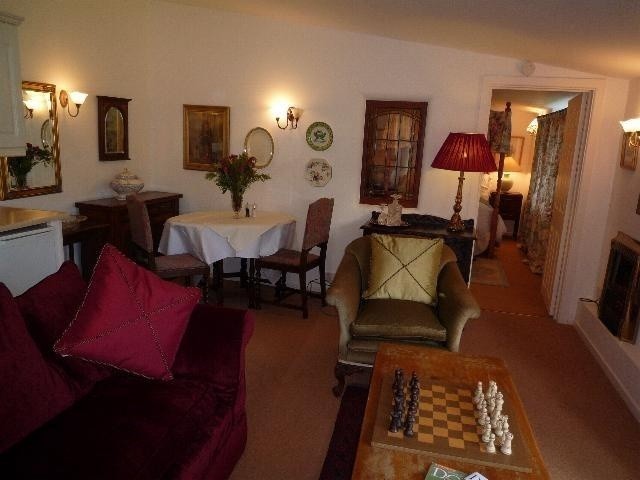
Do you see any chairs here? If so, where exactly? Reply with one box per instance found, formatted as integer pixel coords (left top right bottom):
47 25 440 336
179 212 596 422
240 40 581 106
250 197 335 319
127 194 209 301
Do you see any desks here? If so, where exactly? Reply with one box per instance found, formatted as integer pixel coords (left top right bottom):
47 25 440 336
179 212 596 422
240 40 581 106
166 209 297 307
56 212 111 273
362 215 475 291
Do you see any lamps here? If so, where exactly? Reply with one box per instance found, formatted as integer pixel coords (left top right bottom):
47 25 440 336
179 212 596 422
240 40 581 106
269 94 302 129
495 156 523 192
60 89 88 118
429 132 498 224
618 117 640 145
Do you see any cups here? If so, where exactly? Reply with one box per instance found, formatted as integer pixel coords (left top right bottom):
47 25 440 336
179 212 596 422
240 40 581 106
378 204 388 224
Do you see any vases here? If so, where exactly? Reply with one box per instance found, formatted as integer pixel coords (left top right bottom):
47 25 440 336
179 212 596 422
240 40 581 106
18 173 27 189
231 198 242 220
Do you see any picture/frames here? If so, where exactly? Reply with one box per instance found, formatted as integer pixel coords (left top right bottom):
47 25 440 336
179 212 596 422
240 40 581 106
305 121 334 152
0 81 63 201
358 98 428 209
302 158 334 188
181 104 229 171
620 132 640 171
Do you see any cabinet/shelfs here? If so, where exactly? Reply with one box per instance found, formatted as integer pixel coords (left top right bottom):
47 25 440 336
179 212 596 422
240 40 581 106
0 7 32 158
0 205 64 298
77 180 183 279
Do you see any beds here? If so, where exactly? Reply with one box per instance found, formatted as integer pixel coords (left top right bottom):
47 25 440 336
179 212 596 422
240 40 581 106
462 173 507 258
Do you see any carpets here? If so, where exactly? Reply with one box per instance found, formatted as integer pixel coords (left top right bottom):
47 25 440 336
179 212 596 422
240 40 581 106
468 249 510 288
315 383 369 480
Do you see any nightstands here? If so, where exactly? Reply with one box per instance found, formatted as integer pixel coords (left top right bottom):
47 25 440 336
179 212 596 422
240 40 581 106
486 189 521 241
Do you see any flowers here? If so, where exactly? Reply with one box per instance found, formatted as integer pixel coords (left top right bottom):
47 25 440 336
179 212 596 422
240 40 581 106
3 140 53 178
205 153 270 220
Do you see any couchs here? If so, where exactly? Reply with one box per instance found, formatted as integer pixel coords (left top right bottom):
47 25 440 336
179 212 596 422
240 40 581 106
324 233 482 365
0 260 254 480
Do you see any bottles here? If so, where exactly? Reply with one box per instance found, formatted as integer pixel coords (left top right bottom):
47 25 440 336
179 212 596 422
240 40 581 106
246 202 250 217
252 201 257 218
387 195 402 226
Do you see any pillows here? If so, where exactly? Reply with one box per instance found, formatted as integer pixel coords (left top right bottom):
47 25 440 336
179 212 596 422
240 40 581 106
364 232 443 308
51 243 204 381
474 173 492 204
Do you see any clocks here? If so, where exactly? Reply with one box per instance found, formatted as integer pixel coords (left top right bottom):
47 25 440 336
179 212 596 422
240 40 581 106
97 94 133 162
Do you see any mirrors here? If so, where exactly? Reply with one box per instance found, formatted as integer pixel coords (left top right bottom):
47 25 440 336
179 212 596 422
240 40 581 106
243 127 274 169
